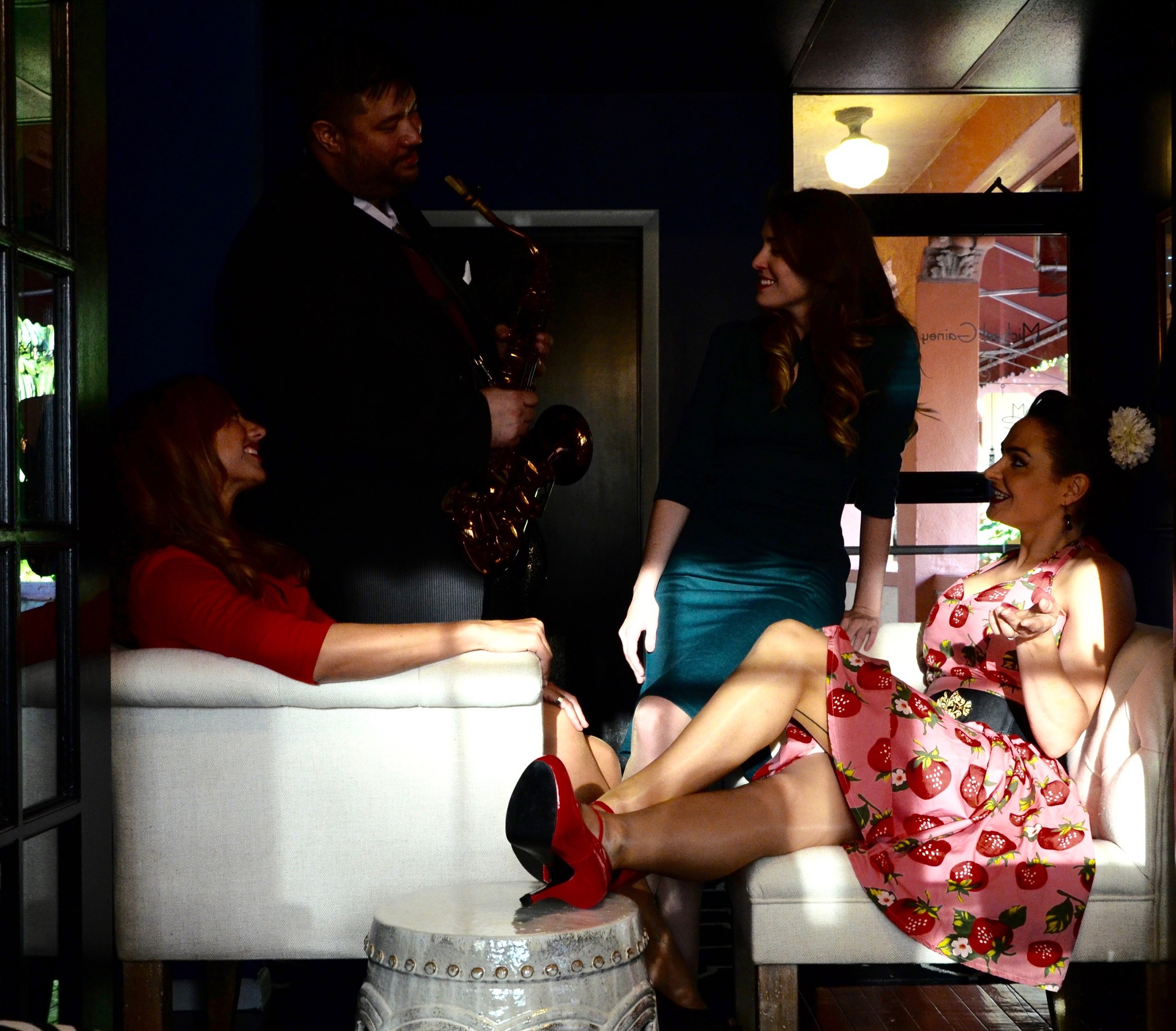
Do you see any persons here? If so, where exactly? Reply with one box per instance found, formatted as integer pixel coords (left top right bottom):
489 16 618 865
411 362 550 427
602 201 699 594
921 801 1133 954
220 55 556 617
506 389 1155 998
615 189 921 976
124 372 734 1031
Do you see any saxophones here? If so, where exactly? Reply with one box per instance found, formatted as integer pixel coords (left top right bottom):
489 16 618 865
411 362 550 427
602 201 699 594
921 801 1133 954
434 162 596 578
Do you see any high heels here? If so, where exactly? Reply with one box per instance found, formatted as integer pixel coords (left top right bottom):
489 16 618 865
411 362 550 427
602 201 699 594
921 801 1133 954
506 754 636 910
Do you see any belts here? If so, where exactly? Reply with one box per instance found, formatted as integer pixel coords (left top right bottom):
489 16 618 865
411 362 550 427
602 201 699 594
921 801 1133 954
932 690 1034 745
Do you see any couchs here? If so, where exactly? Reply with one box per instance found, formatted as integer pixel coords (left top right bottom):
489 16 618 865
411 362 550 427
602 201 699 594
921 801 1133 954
747 621 1176 1031
108 648 546 1031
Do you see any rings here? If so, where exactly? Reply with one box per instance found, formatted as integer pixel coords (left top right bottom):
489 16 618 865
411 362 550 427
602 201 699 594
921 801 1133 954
1007 632 1018 641
555 694 565 708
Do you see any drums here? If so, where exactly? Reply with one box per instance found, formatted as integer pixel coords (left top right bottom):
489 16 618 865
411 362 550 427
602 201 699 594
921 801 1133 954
355 879 659 1031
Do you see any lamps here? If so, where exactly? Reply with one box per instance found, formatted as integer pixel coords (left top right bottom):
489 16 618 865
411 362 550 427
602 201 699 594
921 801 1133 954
824 107 889 188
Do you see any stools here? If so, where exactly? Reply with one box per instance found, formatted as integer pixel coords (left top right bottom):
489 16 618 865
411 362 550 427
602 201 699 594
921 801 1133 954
358 878 660 1031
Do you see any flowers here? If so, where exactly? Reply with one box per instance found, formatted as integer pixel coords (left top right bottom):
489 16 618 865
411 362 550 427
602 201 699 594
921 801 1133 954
1107 406 1157 470
